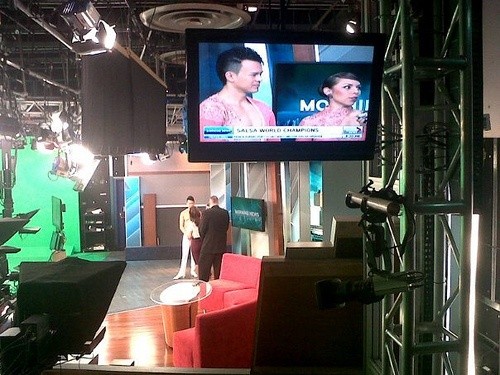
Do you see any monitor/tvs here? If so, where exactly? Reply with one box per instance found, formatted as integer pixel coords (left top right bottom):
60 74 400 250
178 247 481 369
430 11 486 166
230 196 264 233
183 27 390 163
51 196 62 233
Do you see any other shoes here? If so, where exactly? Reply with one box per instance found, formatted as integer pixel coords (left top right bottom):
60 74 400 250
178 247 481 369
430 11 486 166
173 273 185 279
191 272 197 277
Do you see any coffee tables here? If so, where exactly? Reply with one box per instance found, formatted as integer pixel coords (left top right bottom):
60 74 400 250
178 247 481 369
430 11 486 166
149 277 213 348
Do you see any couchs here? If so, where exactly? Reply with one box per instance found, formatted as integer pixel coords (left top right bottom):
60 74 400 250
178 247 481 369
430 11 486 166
172 253 263 370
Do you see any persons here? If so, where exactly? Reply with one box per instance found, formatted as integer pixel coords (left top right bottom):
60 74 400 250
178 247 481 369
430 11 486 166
197 196 230 282
186 206 202 276
295 73 366 142
199 46 280 142
174 196 203 280
